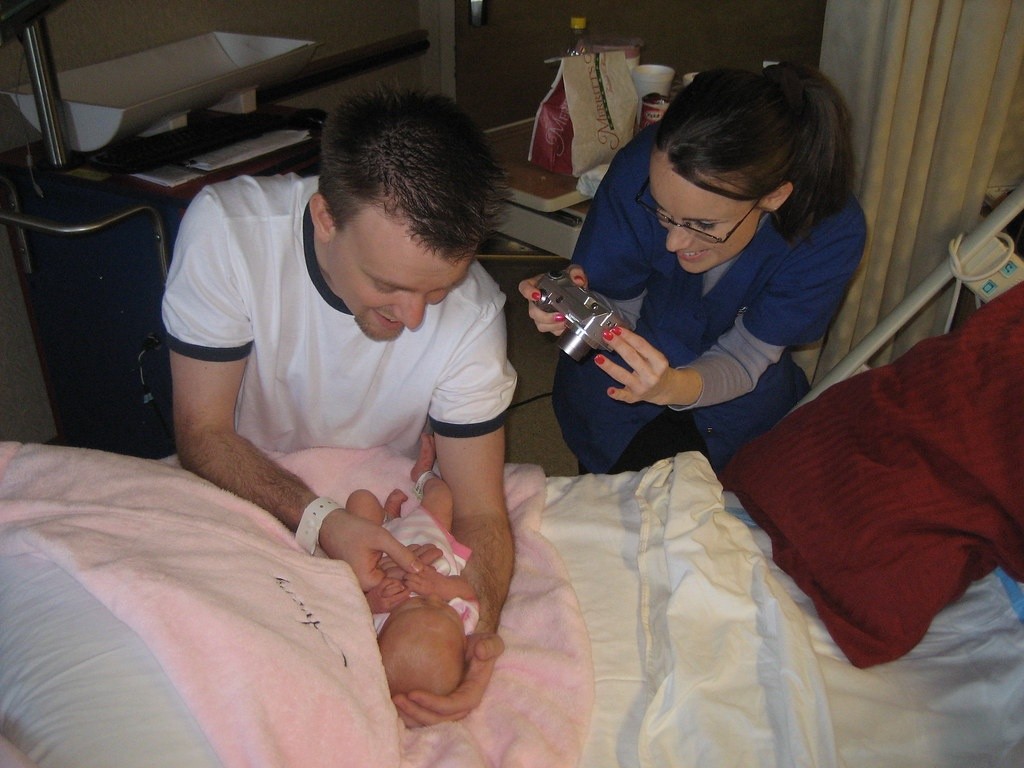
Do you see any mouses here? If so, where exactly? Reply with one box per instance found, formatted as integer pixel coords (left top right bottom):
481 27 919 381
286 108 329 129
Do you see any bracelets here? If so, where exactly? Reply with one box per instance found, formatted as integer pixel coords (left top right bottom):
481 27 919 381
295 496 345 556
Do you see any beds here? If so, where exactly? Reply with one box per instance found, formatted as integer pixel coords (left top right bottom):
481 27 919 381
0 194 1020 768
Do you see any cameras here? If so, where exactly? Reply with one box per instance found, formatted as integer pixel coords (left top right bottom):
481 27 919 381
533 268 630 362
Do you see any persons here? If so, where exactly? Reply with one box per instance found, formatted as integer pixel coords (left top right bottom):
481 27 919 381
519 61 867 475
160 89 518 730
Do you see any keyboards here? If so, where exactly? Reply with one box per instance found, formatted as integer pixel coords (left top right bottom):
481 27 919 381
86 111 289 175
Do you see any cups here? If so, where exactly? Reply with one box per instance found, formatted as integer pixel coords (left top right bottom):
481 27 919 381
632 64 676 124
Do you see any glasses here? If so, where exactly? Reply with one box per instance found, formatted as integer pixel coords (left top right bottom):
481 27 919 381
636 176 760 243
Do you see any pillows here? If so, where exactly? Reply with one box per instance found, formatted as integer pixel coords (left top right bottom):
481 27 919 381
724 284 1022 668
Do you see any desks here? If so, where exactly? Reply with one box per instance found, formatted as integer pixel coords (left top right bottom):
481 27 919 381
123 99 330 224
476 120 631 261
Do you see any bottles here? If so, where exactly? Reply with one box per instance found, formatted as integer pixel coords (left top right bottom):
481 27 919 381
563 17 594 58
